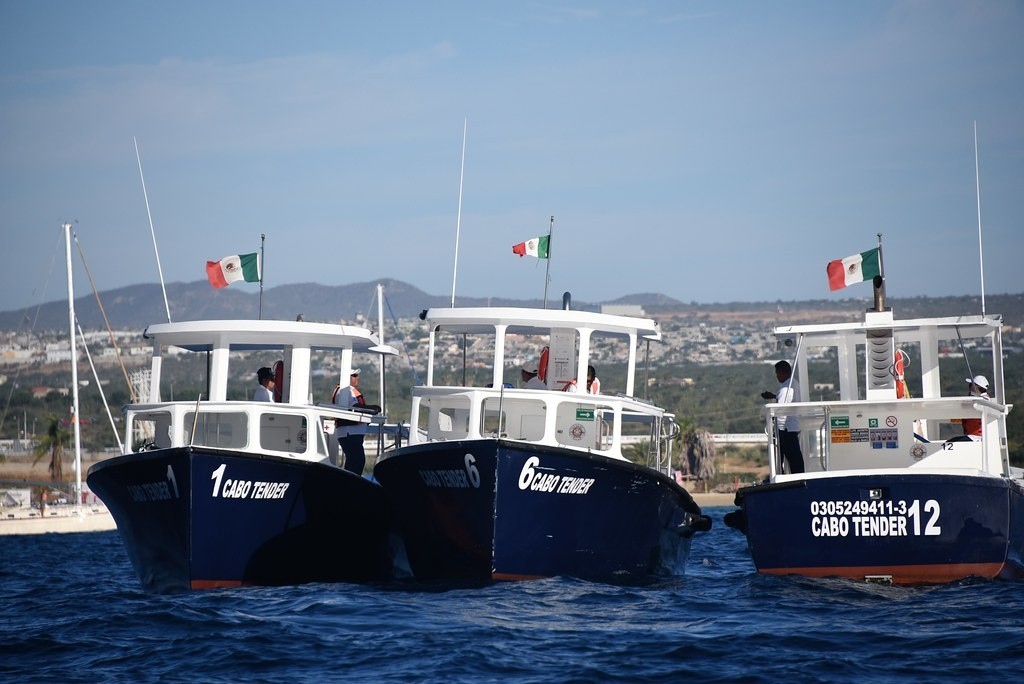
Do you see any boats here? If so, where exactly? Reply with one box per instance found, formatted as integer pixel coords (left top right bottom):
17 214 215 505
372 123 713 590
84 134 407 593
723 118 1024 583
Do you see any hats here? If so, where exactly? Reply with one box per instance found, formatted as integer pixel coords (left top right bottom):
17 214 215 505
965 375 990 390
257 366 276 378
351 367 362 375
519 361 539 374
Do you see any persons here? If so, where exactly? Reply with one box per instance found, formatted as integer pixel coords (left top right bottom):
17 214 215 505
564 365 596 394
40 489 47 517
332 367 379 476
255 366 276 402
760 360 805 474
946 374 991 442
521 358 546 389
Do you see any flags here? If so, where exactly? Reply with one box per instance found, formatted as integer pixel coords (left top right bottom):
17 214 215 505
205 253 262 289
511 234 550 258
827 247 884 291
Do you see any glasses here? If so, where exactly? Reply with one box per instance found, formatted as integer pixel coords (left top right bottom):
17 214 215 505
587 376 594 383
351 373 358 377
